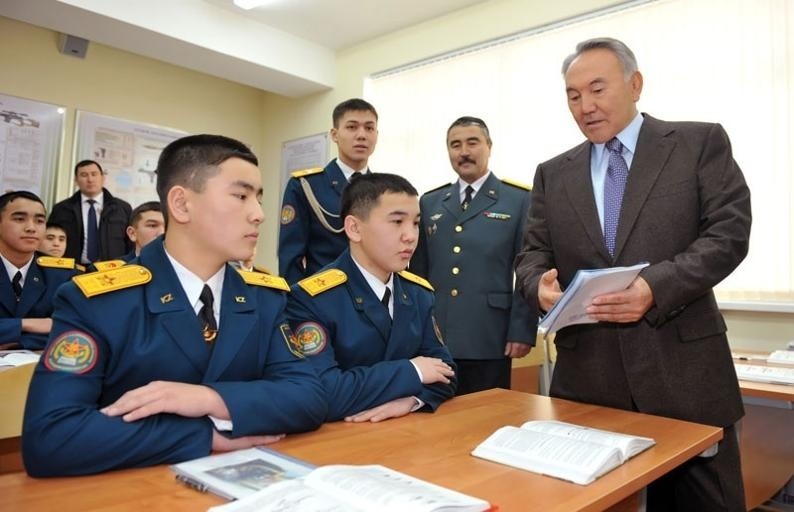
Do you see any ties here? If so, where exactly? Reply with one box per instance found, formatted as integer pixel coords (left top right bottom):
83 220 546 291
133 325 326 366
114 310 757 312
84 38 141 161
196 283 219 354
11 271 23 297
382 286 392 315
461 185 474 211
604 136 629 257
86 199 98 262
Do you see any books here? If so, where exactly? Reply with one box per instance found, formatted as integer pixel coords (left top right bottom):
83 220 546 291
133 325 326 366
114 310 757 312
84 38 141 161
469 418 656 486
209 464 498 512
171 446 321 502
538 260 649 339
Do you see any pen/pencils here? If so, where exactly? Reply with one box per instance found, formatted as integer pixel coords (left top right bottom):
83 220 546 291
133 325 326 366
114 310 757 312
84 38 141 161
175 475 237 502
733 357 751 361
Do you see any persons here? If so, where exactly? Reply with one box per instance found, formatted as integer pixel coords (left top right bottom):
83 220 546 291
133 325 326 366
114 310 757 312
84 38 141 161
409 118 539 396
288 172 458 426
514 36 753 509
278 98 377 286
1 135 328 478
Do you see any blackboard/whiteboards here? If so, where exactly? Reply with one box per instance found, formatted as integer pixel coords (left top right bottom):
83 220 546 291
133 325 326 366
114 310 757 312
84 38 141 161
362 0 794 313
